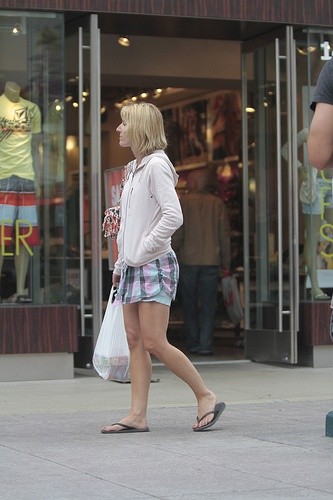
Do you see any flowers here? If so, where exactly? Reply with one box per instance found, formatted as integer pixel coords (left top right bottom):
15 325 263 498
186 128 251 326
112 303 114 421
213 165 241 209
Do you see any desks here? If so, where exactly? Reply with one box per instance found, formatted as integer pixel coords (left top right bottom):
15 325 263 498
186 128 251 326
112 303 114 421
229 231 254 258
0 249 113 301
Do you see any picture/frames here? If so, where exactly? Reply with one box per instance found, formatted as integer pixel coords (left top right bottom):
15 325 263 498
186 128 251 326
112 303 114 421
158 88 257 173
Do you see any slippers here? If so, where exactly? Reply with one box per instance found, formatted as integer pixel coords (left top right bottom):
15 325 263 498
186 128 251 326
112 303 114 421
102 422 149 434
193 401 226 431
17 294 33 303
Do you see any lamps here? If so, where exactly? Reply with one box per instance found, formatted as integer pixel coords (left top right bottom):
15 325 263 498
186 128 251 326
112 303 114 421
156 88 163 93
117 35 131 47
12 23 23 35
221 164 233 177
296 45 316 56
140 92 148 98
115 95 138 108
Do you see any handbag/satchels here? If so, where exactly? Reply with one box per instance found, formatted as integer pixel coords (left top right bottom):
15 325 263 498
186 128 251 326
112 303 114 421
223 270 244 322
92 284 132 382
102 177 126 239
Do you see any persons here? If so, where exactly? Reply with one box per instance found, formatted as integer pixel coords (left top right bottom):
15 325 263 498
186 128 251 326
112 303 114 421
308 56 333 343
172 171 231 356
281 125 333 299
0 81 41 303
100 103 226 433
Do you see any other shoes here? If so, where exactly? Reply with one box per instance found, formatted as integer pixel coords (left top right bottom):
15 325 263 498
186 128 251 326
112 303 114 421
199 350 213 356
188 346 198 354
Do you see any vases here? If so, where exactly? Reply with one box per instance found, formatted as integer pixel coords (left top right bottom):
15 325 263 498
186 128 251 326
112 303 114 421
228 209 242 230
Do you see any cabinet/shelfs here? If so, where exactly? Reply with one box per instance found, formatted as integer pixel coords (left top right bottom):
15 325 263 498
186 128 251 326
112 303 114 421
167 274 306 348
